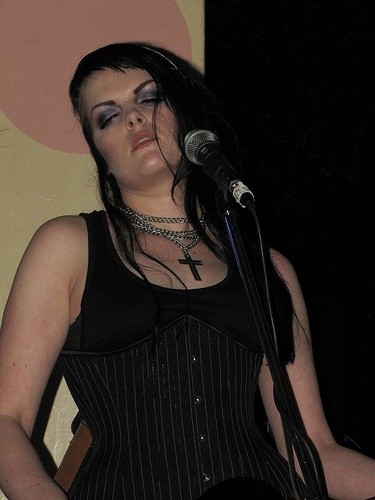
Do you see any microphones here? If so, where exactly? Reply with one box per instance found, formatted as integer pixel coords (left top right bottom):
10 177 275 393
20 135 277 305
182 129 258 215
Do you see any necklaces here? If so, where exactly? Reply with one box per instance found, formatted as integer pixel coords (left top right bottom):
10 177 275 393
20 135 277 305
123 207 211 282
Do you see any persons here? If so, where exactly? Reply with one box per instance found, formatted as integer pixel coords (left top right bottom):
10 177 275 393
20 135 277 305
0 39 375 500
